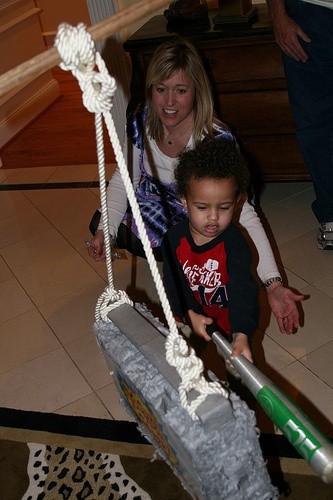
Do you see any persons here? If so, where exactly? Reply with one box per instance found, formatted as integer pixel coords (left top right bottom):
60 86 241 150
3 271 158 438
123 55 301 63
267 0 333 251
87 38 305 334
160 148 260 402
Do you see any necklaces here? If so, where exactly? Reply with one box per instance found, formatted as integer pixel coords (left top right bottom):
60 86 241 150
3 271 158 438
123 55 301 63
163 127 191 145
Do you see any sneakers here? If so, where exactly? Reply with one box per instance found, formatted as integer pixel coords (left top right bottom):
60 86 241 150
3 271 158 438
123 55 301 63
315 222 333 250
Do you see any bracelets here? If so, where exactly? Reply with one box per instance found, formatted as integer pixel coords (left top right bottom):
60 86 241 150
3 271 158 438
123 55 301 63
261 277 282 290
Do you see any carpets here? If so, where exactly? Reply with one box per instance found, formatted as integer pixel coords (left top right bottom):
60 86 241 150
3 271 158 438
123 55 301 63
0 407 333 500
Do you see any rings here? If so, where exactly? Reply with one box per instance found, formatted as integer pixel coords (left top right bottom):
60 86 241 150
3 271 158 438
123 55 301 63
283 317 287 321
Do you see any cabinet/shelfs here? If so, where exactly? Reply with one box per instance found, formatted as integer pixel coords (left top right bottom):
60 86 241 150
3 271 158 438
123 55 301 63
121 3 313 183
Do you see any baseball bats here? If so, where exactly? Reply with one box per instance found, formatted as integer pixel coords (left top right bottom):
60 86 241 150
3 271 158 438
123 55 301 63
205 322 333 485
